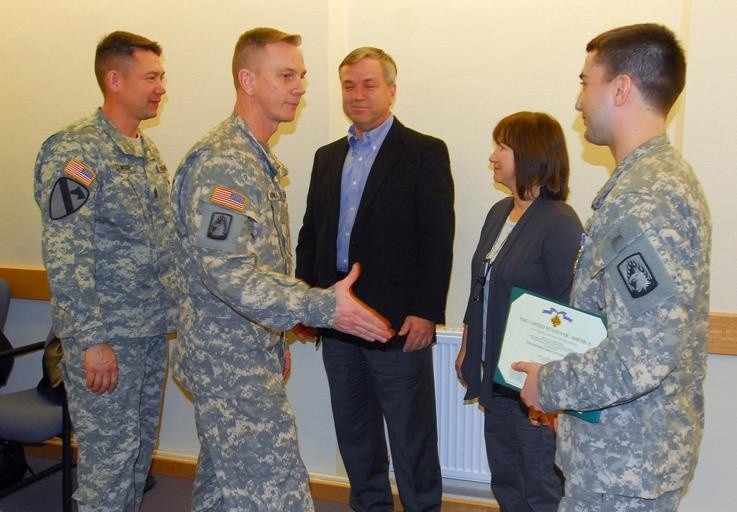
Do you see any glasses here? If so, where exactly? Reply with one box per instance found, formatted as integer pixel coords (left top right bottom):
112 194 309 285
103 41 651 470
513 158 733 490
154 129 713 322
471 258 495 304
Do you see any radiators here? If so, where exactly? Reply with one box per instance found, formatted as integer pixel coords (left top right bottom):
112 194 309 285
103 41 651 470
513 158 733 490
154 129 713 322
384 333 495 500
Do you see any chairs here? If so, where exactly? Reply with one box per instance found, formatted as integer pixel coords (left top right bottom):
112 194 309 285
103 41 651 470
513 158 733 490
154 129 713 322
0 341 156 512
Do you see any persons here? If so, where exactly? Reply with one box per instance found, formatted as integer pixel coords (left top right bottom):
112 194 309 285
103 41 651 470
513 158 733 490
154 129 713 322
454 112 585 512
33 31 172 510
289 48 453 512
511 25 711 512
171 29 393 512
